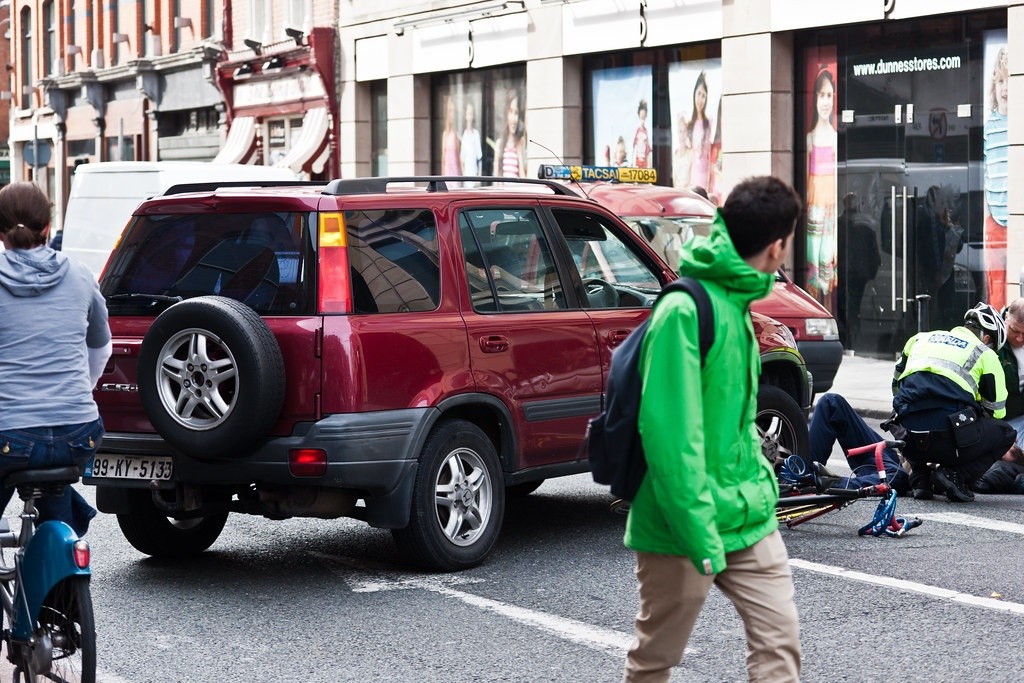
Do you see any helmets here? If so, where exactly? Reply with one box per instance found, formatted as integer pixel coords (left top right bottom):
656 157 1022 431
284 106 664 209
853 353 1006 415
965 302 1007 351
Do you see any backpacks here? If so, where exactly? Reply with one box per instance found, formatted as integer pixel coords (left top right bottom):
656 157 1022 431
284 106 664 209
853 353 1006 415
583 277 714 502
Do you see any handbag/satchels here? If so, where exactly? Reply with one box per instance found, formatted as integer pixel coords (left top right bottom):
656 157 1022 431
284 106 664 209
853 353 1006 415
948 408 982 448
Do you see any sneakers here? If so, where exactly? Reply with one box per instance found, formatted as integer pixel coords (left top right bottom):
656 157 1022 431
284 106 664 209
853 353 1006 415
936 462 975 502
910 473 932 500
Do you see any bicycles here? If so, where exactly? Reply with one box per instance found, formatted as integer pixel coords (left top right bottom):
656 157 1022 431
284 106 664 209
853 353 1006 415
608 437 915 539
1 464 98 683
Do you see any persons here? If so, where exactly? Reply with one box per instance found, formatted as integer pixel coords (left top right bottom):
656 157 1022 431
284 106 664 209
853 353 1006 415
459 99 483 189
709 195 720 207
996 298 1024 453
983 47 1009 314
837 191 881 351
691 185 709 200
0 178 115 538
492 88 526 178
441 95 462 192
622 176 802 683
804 63 837 314
892 185 963 355
798 393 1024 498
605 68 721 193
891 300 1017 502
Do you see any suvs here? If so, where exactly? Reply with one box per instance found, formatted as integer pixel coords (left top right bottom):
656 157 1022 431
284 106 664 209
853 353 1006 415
436 169 839 397
72 171 818 574
